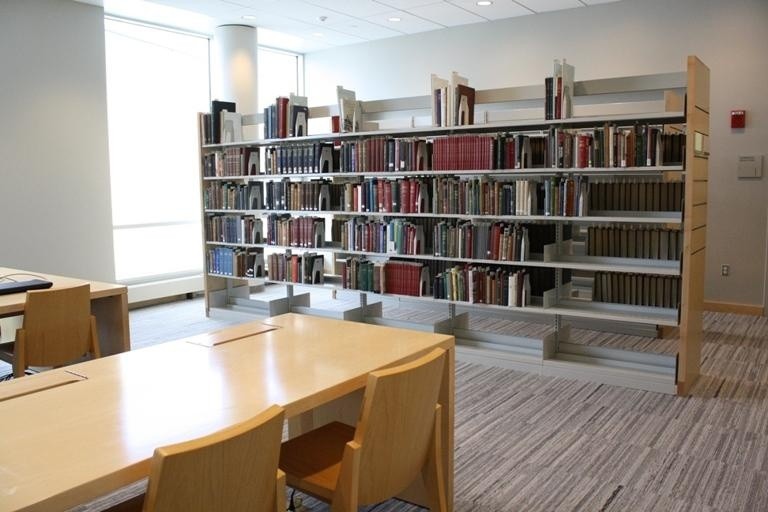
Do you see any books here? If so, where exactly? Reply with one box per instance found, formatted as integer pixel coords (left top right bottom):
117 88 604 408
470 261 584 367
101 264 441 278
340 130 544 173
544 120 684 168
264 92 308 139
341 174 544 216
204 179 264 210
265 140 334 175
266 213 325 248
332 85 361 133
203 101 236 145
331 216 529 262
204 212 265 278
268 249 324 284
561 225 684 260
557 269 682 308
342 256 531 307
545 63 574 120
203 146 260 177
265 177 341 211
431 71 475 127
544 173 684 217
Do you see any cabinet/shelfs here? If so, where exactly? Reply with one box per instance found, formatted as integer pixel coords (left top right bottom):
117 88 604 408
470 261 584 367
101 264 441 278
363 91 454 304
197 109 291 287
454 77 557 314
290 102 363 292
556 69 687 329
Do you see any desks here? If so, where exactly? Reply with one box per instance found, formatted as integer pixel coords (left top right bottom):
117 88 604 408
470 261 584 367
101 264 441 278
0 266 131 356
0 311 456 511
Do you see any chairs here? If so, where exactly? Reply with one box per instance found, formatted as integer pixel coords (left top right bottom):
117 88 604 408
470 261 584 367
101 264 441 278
278 346 450 511
99 403 289 511
0 282 101 379
